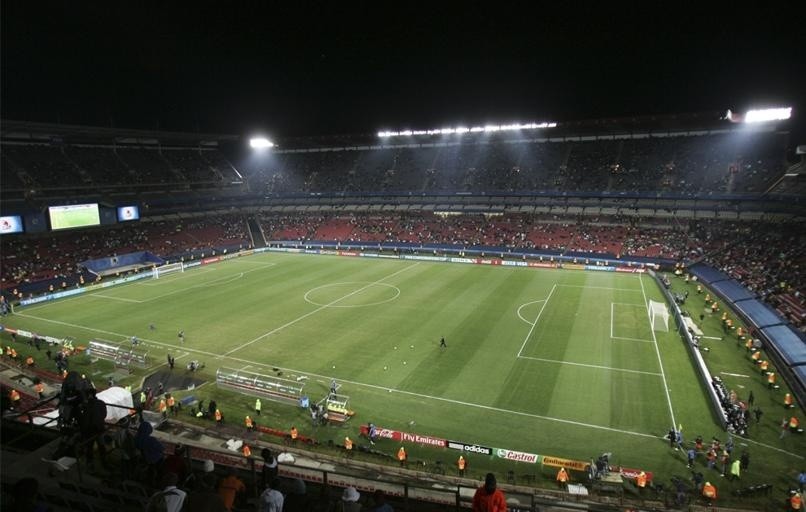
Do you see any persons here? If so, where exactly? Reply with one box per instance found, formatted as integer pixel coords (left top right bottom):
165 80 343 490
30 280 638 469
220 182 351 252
1 133 806 511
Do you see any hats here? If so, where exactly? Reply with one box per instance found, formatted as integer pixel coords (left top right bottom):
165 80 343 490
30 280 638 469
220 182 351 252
341 487 362 501
173 442 186 455
202 459 215 473
